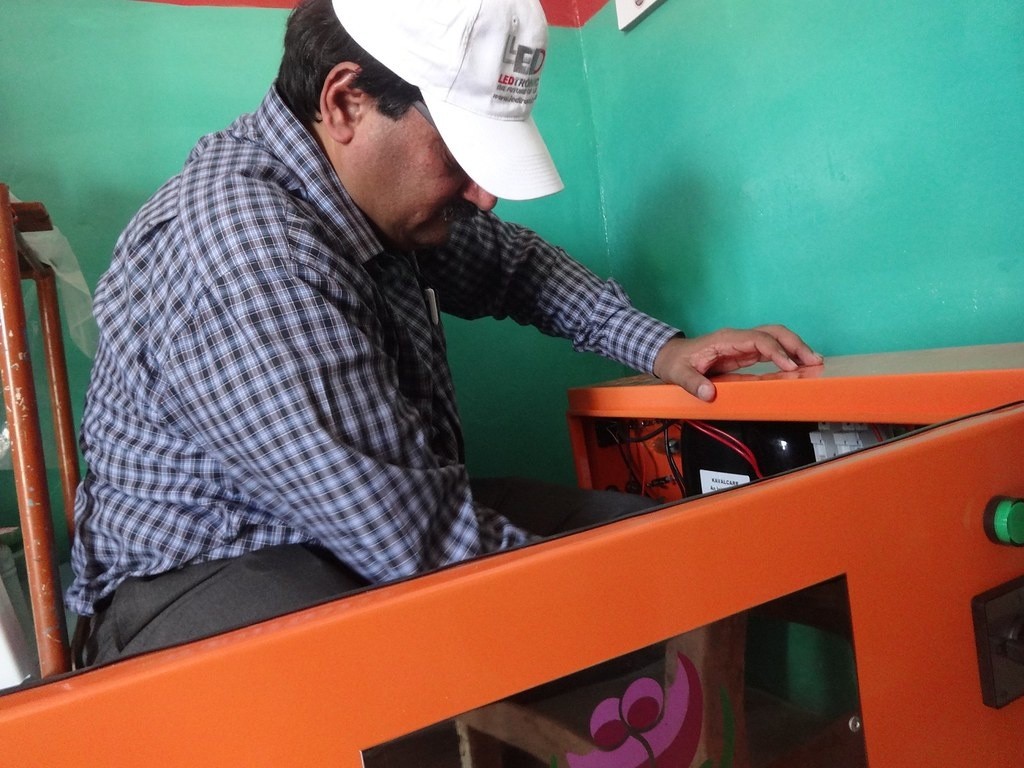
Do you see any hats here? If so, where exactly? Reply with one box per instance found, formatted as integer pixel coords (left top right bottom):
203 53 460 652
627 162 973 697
332 1 565 201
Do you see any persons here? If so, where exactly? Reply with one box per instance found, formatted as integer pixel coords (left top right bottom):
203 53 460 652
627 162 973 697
62 0 824 767
